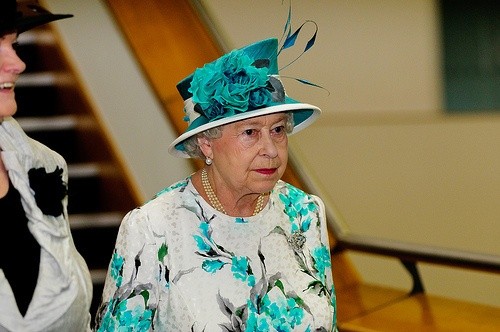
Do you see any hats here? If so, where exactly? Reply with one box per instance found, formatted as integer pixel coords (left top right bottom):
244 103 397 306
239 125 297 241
0 0 75 36
167 1 323 159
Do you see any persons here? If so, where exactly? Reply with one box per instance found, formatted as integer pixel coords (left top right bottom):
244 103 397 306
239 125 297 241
0 0 93 332
97 37 341 332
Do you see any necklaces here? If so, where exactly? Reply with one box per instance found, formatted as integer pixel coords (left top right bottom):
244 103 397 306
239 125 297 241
201 167 265 218
